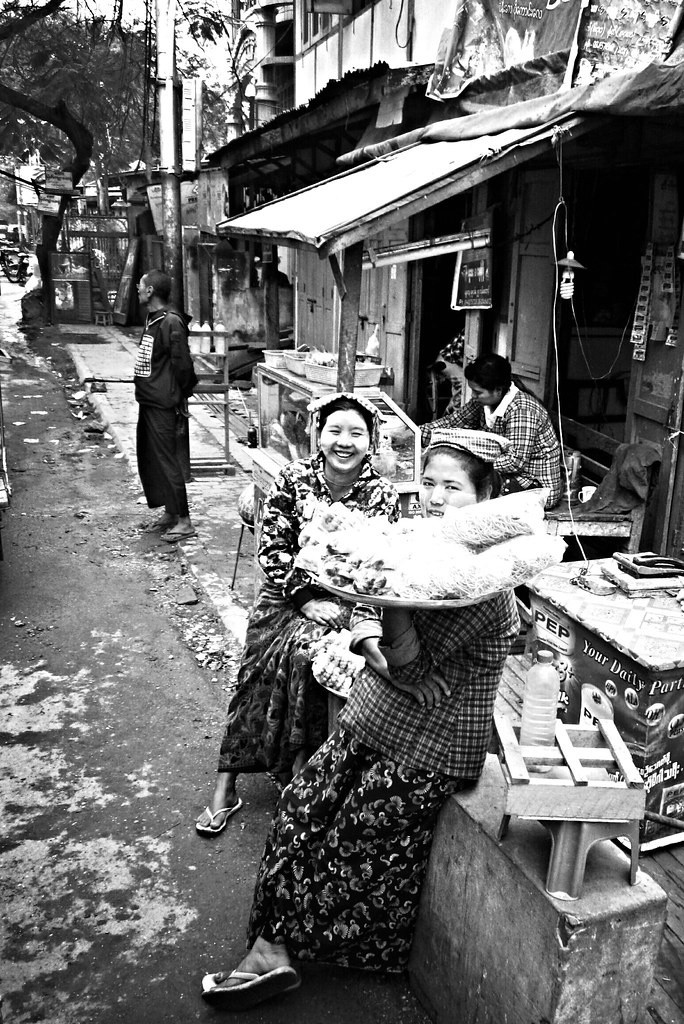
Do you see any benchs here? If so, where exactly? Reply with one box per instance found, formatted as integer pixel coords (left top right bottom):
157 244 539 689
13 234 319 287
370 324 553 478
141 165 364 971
541 411 664 554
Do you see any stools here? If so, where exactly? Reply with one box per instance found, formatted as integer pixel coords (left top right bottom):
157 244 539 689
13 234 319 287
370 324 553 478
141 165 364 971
496 813 641 901
95 311 111 326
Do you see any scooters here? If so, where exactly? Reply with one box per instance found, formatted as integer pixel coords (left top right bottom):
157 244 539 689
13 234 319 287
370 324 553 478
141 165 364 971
0 246 29 285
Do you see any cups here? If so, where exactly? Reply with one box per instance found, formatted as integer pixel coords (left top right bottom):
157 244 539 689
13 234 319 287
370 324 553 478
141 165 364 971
381 450 399 477
578 486 597 503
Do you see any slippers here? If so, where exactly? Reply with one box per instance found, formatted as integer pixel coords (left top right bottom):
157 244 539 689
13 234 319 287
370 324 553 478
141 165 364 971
146 519 178 533
201 961 305 1009
161 531 198 542
196 793 243 834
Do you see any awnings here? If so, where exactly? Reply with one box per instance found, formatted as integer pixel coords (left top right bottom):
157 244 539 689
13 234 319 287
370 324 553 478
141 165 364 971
213 108 603 391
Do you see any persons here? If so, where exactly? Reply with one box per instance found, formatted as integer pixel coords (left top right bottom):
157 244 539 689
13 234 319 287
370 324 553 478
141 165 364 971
135 269 198 543
203 429 518 1004
390 354 562 510
12 228 19 243
197 392 398 834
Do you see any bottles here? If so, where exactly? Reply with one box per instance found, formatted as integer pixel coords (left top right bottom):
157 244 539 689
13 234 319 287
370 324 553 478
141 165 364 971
375 453 380 472
202 321 211 353
213 319 225 354
520 650 560 773
188 321 201 353
280 410 293 446
247 425 257 448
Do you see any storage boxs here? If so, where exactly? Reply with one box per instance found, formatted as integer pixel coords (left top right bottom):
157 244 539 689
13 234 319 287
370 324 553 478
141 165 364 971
262 349 385 386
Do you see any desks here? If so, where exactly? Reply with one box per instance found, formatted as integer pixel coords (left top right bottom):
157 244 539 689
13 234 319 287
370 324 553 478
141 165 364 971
525 550 684 859
